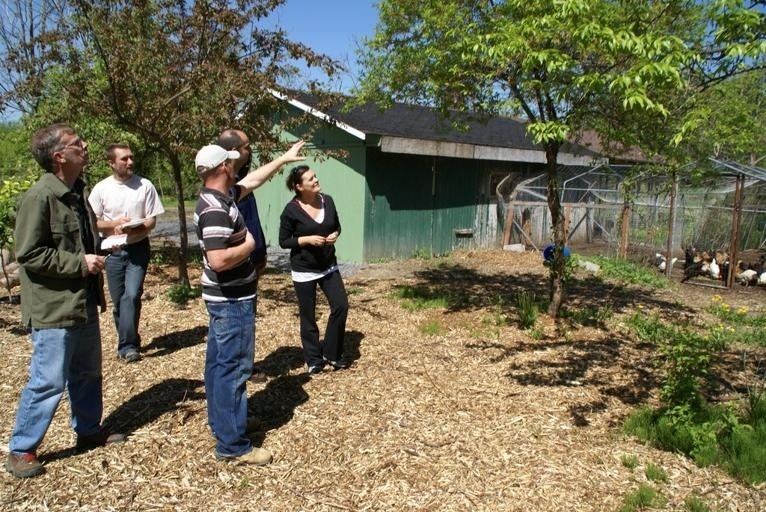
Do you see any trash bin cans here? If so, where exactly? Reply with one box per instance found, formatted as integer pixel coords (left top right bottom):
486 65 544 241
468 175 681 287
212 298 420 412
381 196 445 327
544 243 570 261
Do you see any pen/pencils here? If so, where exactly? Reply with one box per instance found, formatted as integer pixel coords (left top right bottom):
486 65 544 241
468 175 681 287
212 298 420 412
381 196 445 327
104 253 112 261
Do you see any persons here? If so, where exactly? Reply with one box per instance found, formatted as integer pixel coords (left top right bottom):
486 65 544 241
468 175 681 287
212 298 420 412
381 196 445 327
88 143 165 364
216 128 269 384
277 164 350 375
4 123 128 479
193 138 308 464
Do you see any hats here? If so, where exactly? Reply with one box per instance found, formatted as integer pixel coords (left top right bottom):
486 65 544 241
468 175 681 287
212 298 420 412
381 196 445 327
195 145 240 173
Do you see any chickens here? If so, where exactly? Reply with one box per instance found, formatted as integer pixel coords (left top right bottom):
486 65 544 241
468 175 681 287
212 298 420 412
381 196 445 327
680 249 766 288
655 253 678 274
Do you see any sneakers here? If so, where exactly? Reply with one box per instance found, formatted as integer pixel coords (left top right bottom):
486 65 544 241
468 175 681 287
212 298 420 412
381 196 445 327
216 417 272 464
77 429 124 443
125 352 140 361
6 454 45 477
304 355 350 374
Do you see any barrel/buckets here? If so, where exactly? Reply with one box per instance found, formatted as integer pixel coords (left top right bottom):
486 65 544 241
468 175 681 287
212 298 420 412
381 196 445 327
542 243 571 264
